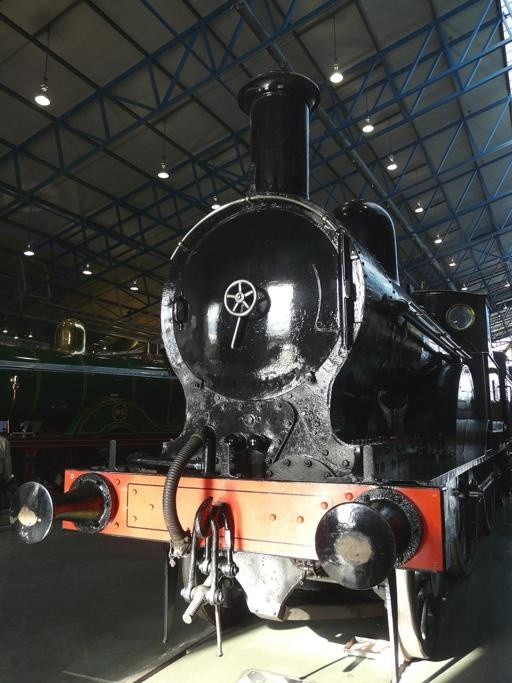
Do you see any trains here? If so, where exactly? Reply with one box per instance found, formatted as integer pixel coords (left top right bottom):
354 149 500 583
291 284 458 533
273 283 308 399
6 65 512 662
1 317 187 506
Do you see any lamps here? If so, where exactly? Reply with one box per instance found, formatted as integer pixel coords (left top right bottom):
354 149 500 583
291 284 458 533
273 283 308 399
413 168 468 291
157 117 170 179
329 10 399 172
211 159 222 210
24 195 140 291
34 23 51 106
